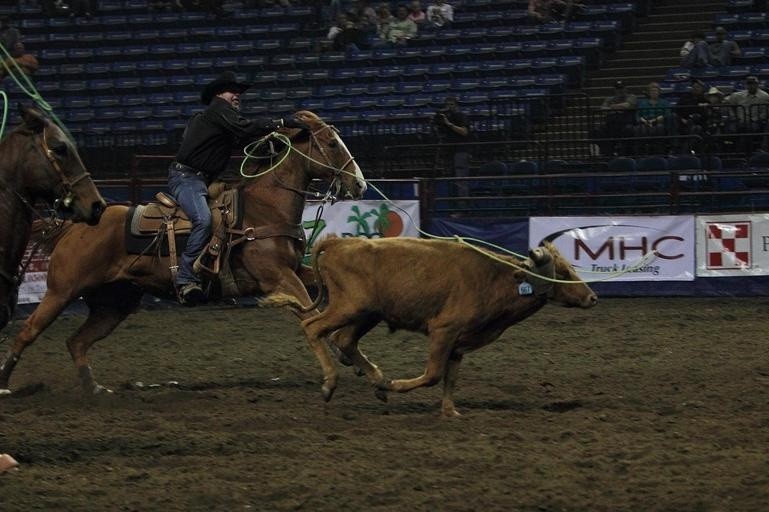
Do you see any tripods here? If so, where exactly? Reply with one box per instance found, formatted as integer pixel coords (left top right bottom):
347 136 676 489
429 137 459 212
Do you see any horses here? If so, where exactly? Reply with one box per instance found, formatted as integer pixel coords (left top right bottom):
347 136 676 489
0 109 388 406
0 101 109 476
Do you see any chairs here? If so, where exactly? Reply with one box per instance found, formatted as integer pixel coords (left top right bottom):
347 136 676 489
0 1 769 156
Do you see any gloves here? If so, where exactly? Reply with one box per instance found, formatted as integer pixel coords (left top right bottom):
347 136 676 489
280 115 310 130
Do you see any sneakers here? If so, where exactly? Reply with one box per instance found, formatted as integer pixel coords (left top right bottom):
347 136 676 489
180 282 204 304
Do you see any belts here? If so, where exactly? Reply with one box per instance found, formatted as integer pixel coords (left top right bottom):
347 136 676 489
172 161 207 176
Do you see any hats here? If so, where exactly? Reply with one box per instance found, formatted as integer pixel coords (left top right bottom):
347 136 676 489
614 75 760 96
201 71 249 106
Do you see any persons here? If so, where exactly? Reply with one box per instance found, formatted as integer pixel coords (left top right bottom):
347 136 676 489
326 0 586 52
599 26 769 158
166 83 309 306
0 22 38 86
432 95 468 135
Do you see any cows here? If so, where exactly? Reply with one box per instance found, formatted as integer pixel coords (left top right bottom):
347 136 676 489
254 234 599 418
